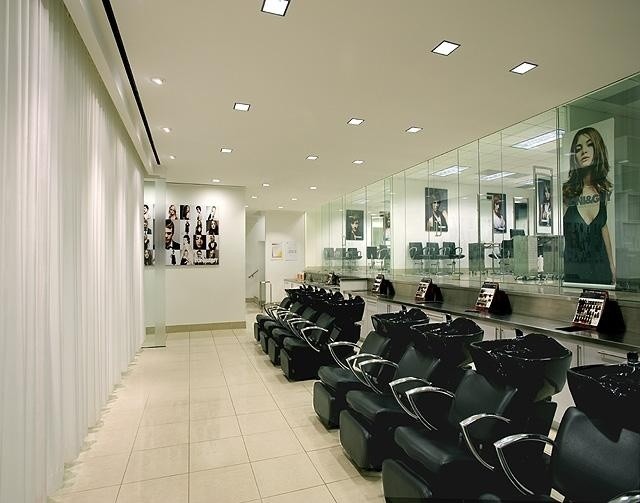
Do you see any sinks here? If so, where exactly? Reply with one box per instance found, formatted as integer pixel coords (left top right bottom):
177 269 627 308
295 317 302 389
372 308 430 351
410 316 483 364
284 284 366 322
470 333 571 404
568 364 640 442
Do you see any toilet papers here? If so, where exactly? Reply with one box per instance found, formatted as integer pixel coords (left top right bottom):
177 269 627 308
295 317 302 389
538 256 544 273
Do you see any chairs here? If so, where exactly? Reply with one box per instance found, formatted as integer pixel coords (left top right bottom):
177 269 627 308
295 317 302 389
409 240 466 277
313 305 640 502
253 288 365 381
488 229 526 276
323 244 390 271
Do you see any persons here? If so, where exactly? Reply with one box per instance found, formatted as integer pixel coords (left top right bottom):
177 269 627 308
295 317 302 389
565 126 615 284
347 180 554 239
145 205 218 265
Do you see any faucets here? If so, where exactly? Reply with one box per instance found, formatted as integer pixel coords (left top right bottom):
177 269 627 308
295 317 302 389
446 313 452 324
400 304 408 316
626 350 640 372
514 327 525 341
